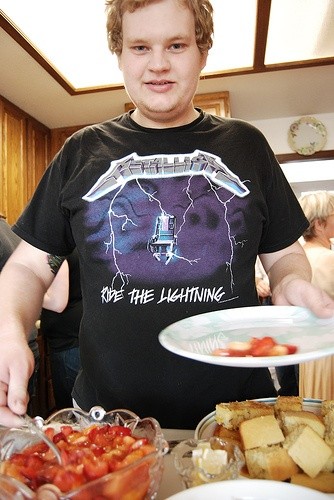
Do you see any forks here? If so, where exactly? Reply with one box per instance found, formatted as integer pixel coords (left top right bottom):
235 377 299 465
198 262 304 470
13 410 68 467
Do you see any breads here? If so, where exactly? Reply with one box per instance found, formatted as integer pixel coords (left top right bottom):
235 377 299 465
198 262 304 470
191 396 334 494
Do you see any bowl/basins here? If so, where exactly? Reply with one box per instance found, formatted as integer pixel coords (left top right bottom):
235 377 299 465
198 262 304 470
0 405 170 500
193 395 334 479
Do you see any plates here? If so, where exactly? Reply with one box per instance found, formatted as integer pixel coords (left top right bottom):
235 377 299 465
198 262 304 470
158 305 334 369
288 118 326 154
164 478 334 500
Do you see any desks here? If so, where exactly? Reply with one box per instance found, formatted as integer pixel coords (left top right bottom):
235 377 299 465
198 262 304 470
155 428 195 500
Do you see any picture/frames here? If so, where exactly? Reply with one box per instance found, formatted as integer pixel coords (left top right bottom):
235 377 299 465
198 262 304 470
124 90 232 119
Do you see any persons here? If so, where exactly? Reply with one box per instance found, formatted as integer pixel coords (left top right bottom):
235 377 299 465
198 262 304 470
254 254 273 306
0 0 334 430
0 216 23 272
297 189 334 403
41 247 83 410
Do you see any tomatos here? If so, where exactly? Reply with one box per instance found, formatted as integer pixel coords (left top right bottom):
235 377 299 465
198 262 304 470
0 425 155 499
211 337 297 357
